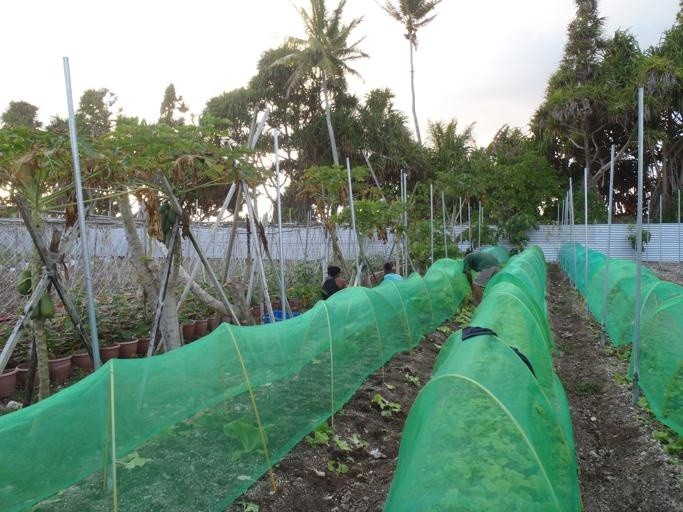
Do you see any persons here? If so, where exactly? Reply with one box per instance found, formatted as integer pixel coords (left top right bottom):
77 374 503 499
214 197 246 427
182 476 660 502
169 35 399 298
383 263 404 281
321 266 347 299
463 250 503 305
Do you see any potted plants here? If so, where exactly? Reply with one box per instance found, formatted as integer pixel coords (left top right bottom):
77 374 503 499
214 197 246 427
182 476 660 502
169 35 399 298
0 259 413 399
